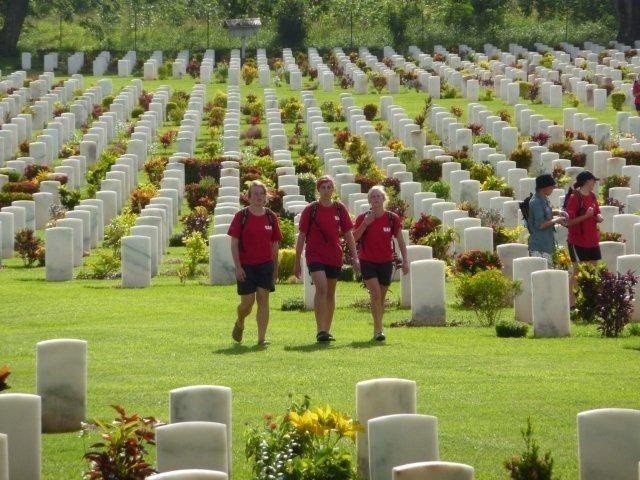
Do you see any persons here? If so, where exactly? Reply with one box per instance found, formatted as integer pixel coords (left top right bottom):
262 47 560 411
561 170 605 319
632 75 640 117
352 184 410 342
226 181 284 346
527 173 569 270
295 176 362 343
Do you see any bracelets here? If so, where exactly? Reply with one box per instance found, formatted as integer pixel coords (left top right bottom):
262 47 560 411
354 258 360 264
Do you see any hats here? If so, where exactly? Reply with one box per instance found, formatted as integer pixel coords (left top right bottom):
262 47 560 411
316 175 334 192
576 170 600 184
536 173 556 188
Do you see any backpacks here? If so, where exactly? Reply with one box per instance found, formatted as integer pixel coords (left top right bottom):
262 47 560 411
519 192 533 234
560 187 573 228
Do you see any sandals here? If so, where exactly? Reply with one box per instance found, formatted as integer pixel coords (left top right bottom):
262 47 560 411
258 340 270 345
231 322 245 342
316 331 333 341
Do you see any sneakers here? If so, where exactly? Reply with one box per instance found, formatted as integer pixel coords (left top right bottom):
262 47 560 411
374 332 385 341
